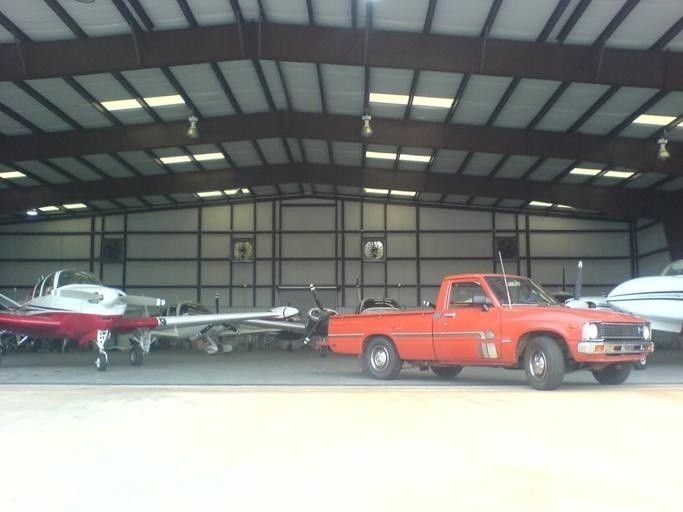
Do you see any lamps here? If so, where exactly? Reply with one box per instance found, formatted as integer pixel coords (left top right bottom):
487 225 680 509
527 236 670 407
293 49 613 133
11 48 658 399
359 108 374 139
186 109 199 138
657 132 671 161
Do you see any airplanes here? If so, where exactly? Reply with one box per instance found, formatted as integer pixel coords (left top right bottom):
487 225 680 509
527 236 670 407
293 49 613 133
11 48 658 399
565 259 683 349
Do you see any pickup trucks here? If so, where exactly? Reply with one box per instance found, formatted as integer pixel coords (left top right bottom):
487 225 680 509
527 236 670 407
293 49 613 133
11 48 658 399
329 273 654 389
